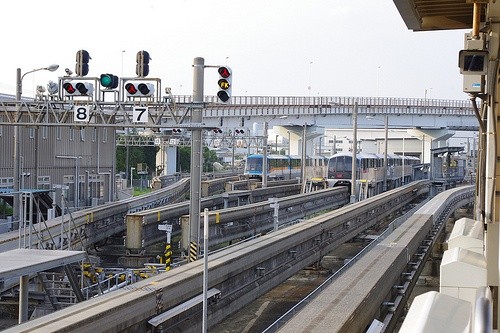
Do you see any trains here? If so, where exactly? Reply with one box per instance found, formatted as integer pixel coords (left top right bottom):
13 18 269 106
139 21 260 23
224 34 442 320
242 153 329 180
326 153 422 188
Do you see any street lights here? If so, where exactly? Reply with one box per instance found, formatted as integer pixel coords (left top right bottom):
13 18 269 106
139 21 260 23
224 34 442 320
13 63 60 228
326 100 359 201
287 121 309 182
365 112 390 189
261 114 289 188
410 134 426 164
324 135 337 154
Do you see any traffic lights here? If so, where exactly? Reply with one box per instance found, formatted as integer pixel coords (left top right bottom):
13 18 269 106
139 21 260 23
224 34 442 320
123 82 154 98
216 64 233 106
58 79 94 99
101 71 118 88
